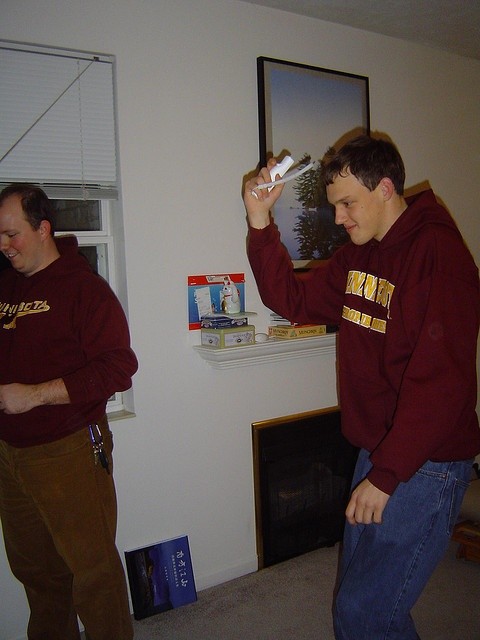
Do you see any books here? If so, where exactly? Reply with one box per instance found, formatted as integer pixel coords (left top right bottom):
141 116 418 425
125 535 197 621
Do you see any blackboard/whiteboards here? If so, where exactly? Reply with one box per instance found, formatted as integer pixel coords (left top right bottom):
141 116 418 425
251 404 362 572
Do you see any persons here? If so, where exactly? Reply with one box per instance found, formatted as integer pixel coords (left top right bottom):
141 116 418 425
0 181 137 640
244 130 480 638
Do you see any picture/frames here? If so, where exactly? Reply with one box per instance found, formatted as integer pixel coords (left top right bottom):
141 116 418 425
258 55 371 275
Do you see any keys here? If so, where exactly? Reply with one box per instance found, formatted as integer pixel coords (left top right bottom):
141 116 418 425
88 425 115 479
